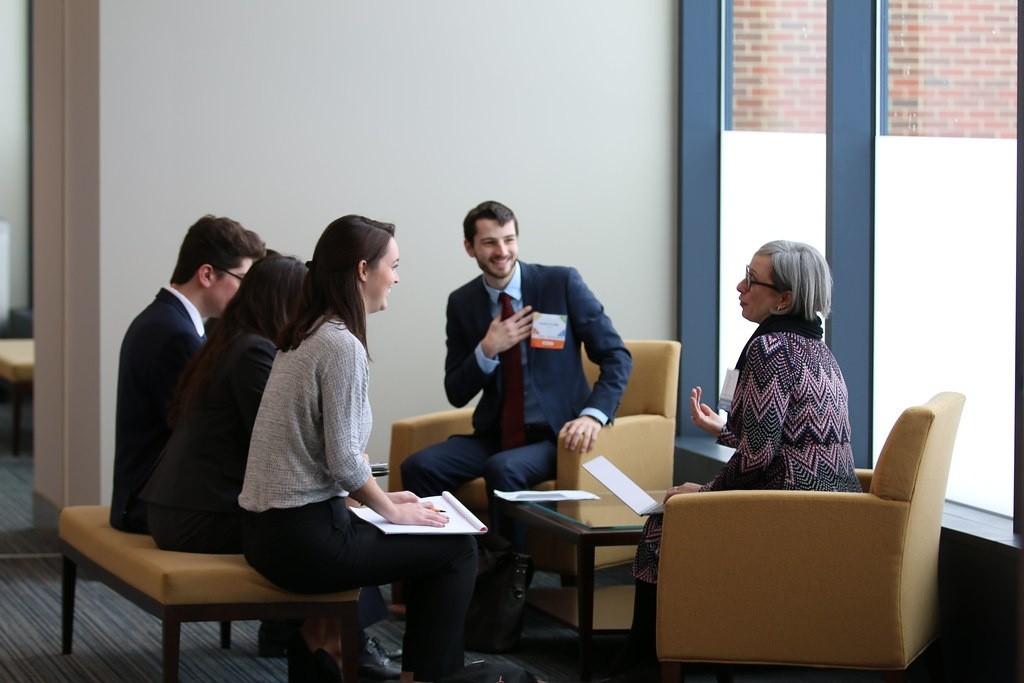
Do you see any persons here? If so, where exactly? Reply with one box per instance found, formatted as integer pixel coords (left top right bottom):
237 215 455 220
606 240 863 677
240 215 476 683
110 216 266 535
145 257 401 681
389 201 632 620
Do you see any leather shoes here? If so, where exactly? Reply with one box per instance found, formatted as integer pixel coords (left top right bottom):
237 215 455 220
342 630 402 683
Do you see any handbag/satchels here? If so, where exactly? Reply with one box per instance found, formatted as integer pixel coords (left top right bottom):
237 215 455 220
463 534 535 655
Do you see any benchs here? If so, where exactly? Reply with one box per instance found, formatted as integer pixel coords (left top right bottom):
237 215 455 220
58 504 360 683
1 337 36 458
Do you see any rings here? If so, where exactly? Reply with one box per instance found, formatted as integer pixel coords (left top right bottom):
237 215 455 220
691 416 695 420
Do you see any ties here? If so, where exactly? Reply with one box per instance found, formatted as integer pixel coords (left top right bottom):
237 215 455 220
498 292 526 451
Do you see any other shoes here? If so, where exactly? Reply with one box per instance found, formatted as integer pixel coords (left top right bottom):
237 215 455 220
258 622 287 657
600 671 631 683
389 603 406 621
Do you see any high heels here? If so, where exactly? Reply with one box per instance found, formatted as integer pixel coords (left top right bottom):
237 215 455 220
288 633 344 683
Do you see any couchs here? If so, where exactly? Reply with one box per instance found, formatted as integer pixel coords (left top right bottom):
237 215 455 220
388 340 681 588
655 392 966 683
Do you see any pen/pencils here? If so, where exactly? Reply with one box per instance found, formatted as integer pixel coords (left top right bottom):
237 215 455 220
432 508 446 512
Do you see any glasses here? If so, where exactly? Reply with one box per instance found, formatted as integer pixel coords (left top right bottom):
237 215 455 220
746 264 782 293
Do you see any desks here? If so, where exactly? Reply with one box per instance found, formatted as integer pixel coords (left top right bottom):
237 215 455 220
489 489 668 683
